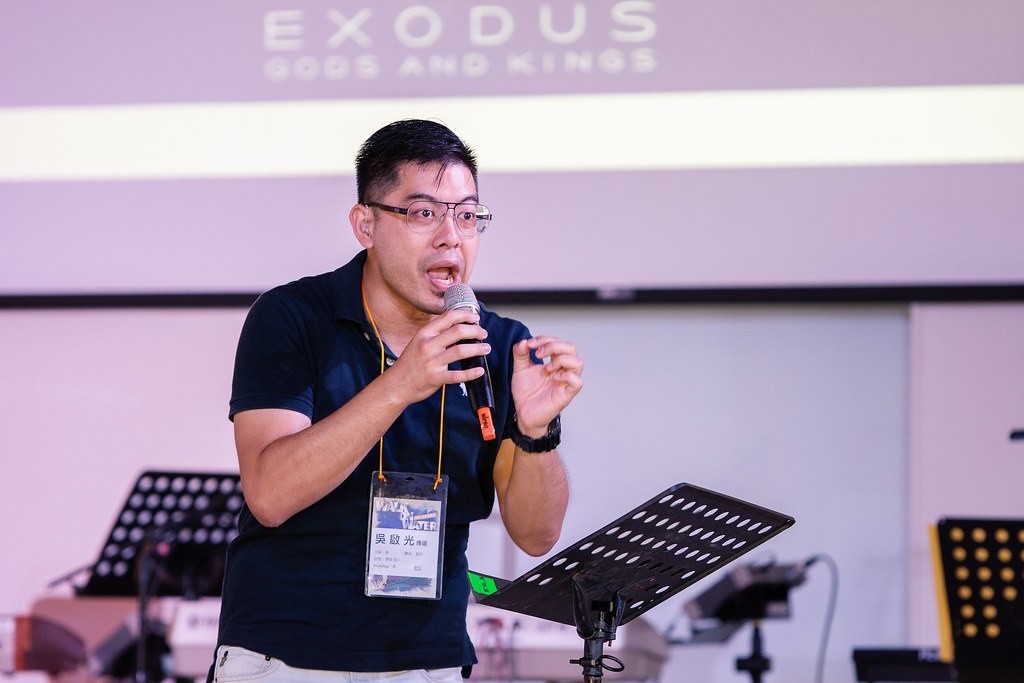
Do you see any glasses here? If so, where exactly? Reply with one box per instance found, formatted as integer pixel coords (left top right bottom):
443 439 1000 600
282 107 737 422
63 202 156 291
364 201 493 237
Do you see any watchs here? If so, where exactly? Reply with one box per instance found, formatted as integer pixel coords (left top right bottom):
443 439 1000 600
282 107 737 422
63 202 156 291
510 413 561 453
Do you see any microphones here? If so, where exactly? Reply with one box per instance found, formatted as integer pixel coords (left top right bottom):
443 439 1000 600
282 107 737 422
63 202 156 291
443 284 497 441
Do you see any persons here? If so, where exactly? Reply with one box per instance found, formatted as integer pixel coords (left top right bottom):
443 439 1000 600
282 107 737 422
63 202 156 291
206 118 584 683
